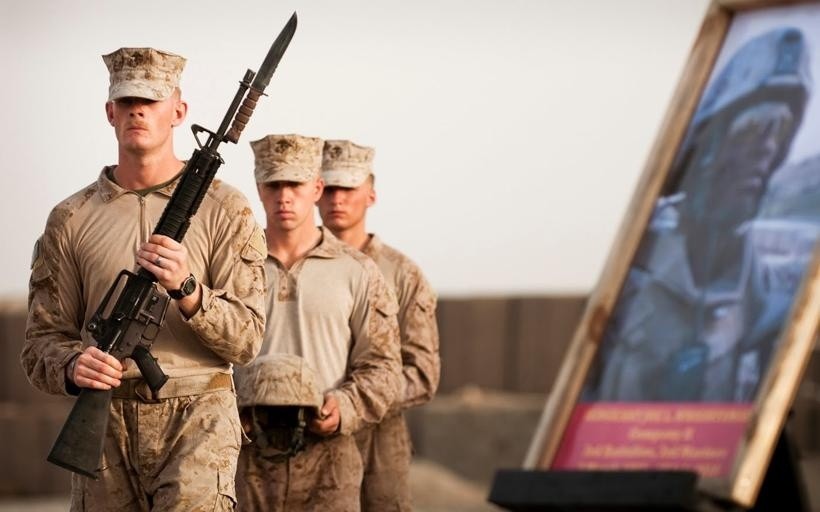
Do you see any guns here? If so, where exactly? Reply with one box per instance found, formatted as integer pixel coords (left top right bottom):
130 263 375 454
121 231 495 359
46 11 298 479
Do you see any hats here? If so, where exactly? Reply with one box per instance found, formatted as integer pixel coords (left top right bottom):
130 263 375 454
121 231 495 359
101 46 187 101
249 132 325 184
324 140 376 188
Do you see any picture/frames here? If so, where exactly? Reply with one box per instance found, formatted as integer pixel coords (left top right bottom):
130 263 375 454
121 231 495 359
521 1 818 508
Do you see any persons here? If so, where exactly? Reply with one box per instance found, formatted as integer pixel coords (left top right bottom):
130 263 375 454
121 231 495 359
317 141 439 512
578 28 812 404
19 48 267 512
232 136 400 512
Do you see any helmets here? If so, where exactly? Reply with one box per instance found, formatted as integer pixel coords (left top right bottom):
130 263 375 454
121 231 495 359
232 354 322 463
694 27 812 122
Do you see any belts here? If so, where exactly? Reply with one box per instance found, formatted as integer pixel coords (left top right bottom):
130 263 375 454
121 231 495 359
111 373 232 404
